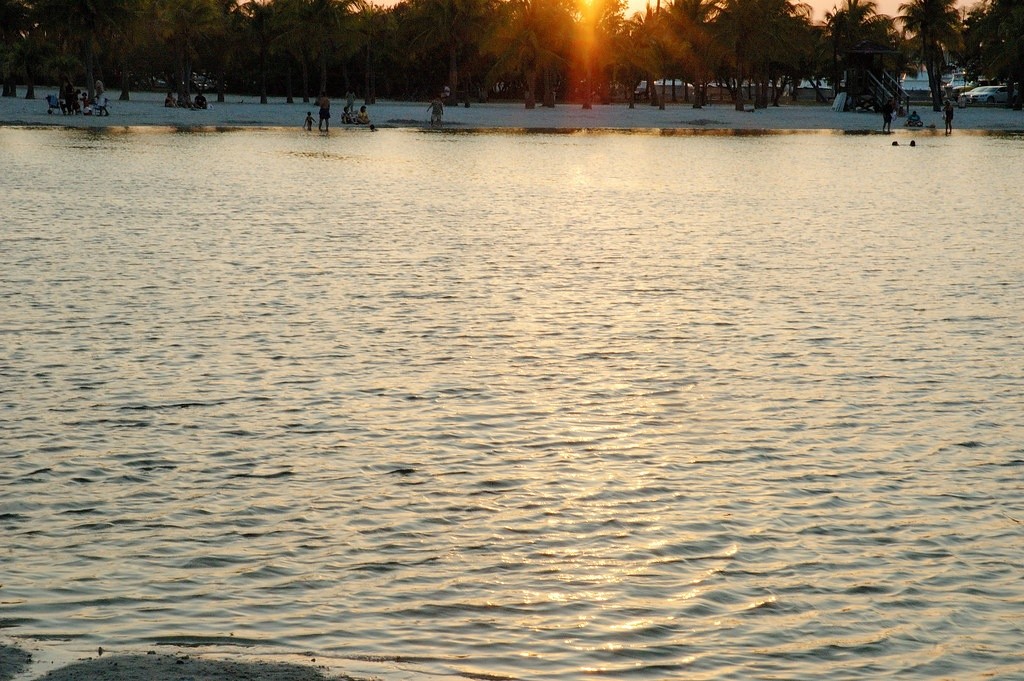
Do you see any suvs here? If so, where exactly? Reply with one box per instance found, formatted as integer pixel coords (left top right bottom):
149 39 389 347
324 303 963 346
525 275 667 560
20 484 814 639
951 78 1001 102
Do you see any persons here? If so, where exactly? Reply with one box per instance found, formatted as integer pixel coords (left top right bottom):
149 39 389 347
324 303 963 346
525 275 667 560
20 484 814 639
942 100 953 135
165 91 207 110
907 111 923 127
305 110 316 130
318 91 330 130
59 75 110 116
427 95 443 127
883 100 893 133
341 86 371 124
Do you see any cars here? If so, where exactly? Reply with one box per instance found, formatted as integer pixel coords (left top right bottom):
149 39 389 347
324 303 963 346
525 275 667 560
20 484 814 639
958 86 990 103
970 85 1019 104
